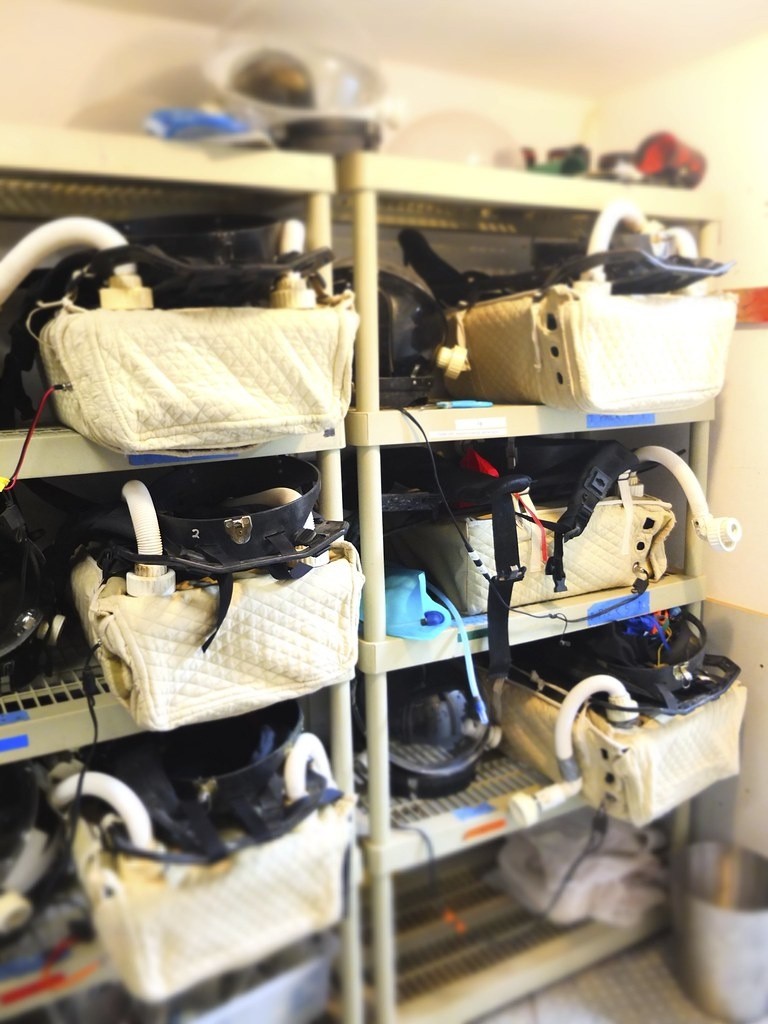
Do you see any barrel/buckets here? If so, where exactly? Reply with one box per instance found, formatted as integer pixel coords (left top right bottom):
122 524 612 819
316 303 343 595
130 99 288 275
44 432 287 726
667 838 768 1023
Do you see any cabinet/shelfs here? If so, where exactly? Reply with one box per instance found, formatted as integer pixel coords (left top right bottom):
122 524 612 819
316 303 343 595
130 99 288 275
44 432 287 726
336 141 723 1024
0 125 369 1024
